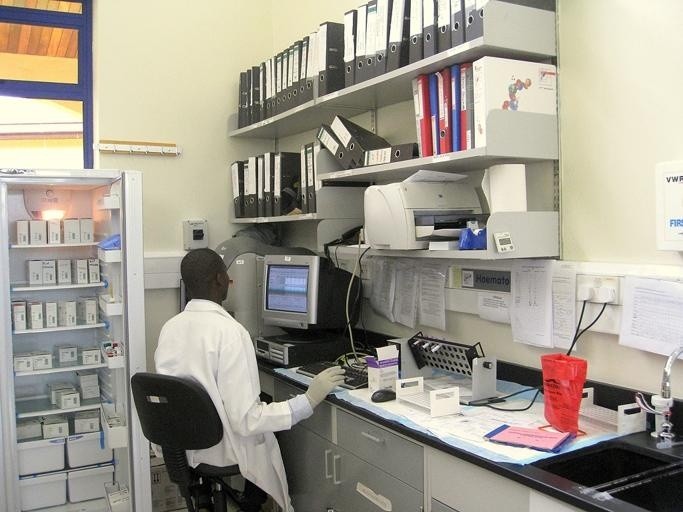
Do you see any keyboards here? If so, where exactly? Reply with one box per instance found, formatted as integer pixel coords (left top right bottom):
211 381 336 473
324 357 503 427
296 360 368 390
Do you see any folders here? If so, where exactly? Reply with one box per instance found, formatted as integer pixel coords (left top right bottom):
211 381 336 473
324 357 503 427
237 0 337 128
231 140 319 218
361 142 418 166
316 124 352 170
329 115 391 167
340 0 560 85
409 62 480 159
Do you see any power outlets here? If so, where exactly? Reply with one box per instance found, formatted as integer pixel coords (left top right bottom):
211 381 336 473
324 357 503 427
576 273 621 306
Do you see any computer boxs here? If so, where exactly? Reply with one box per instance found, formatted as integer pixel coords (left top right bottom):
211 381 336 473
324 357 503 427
256 334 348 369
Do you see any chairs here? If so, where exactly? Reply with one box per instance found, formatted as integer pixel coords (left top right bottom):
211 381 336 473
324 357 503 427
131 372 243 512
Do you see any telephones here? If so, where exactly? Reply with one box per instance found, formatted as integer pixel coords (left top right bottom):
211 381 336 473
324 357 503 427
343 226 361 246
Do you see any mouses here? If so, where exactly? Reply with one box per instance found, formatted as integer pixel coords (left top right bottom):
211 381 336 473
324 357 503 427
372 388 394 404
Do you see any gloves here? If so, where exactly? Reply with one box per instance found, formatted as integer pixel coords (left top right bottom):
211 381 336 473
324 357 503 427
305 365 345 409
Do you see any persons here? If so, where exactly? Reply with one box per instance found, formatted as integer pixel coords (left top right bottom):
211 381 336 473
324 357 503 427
154 247 346 512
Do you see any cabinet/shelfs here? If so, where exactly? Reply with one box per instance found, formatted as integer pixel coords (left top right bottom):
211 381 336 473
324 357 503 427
227 0 563 260
273 378 433 512
427 444 583 512
0 166 153 512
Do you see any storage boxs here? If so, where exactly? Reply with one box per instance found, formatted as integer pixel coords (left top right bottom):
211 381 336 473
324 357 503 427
67 432 115 468
17 437 66 477
150 449 197 512
68 462 115 505
19 471 67 512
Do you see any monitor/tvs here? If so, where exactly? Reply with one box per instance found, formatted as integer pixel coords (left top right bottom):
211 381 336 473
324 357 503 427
261 253 363 344
